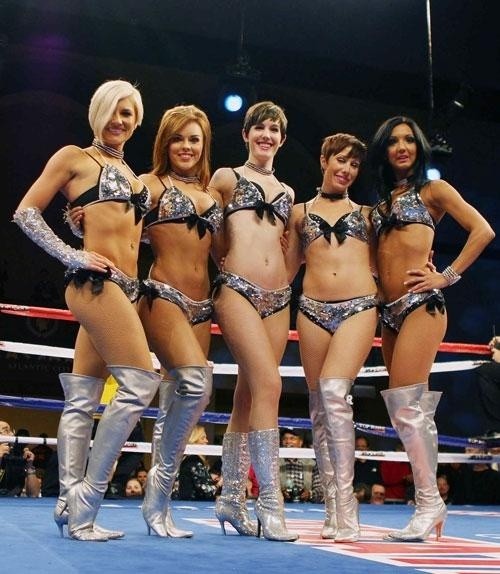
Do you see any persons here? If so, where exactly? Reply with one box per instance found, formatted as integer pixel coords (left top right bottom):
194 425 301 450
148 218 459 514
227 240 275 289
219 134 438 543
10 79 152 541
62 104 291 538
141 100 301 543
369 116 497 542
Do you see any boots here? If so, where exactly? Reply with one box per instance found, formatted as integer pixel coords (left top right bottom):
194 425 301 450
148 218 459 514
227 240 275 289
140 363 214 540
214 430 267 538
379 380 449 545
245 427 301 544
52 363 166 544
308 375 363 546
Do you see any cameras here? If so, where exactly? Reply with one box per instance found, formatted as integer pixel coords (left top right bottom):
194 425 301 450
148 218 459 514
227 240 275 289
6 427 31 462
286 484 304 503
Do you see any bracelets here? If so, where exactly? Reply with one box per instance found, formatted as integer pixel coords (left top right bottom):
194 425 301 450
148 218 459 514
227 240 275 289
442 266 462 286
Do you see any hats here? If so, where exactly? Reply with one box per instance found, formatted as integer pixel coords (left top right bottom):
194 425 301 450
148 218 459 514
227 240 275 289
279 427 303 436
